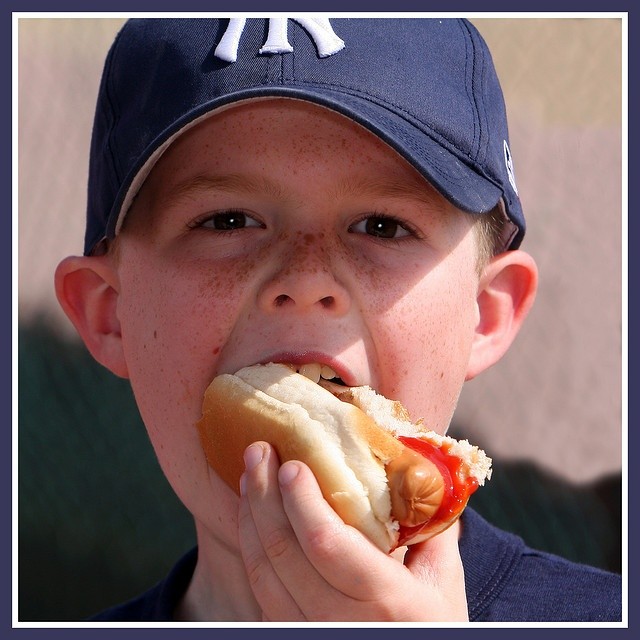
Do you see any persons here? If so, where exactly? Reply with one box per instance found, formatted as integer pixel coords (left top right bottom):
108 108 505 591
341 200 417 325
53 15 619 622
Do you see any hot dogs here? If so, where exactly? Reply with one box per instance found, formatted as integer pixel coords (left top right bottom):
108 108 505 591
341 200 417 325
198 359 493 556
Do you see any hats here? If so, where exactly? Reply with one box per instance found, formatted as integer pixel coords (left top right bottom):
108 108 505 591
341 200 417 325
85 18 525 257
